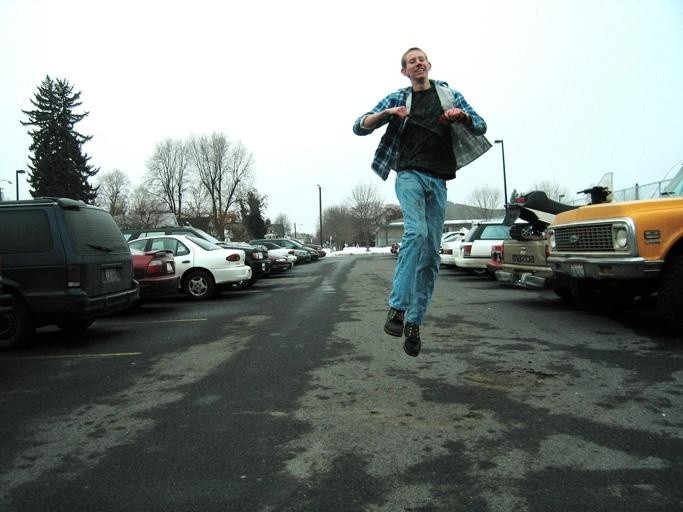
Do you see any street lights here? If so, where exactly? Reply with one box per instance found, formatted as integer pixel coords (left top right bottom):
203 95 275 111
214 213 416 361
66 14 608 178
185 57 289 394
317 184 322 247
16 171 25 199
495 139 508 211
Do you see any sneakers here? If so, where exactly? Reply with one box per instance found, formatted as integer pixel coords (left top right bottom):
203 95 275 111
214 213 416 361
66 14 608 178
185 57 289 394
384 306 421 357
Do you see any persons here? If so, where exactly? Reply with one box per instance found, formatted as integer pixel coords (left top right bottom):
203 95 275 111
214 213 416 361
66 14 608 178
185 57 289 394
351 46 487 356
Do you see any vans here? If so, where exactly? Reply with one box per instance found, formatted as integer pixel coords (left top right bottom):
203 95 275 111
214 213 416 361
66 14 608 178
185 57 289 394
1 198 139 351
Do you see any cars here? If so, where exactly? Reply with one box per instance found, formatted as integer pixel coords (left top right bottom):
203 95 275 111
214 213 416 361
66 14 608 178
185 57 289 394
120 229 326 300
130 247 180 300
